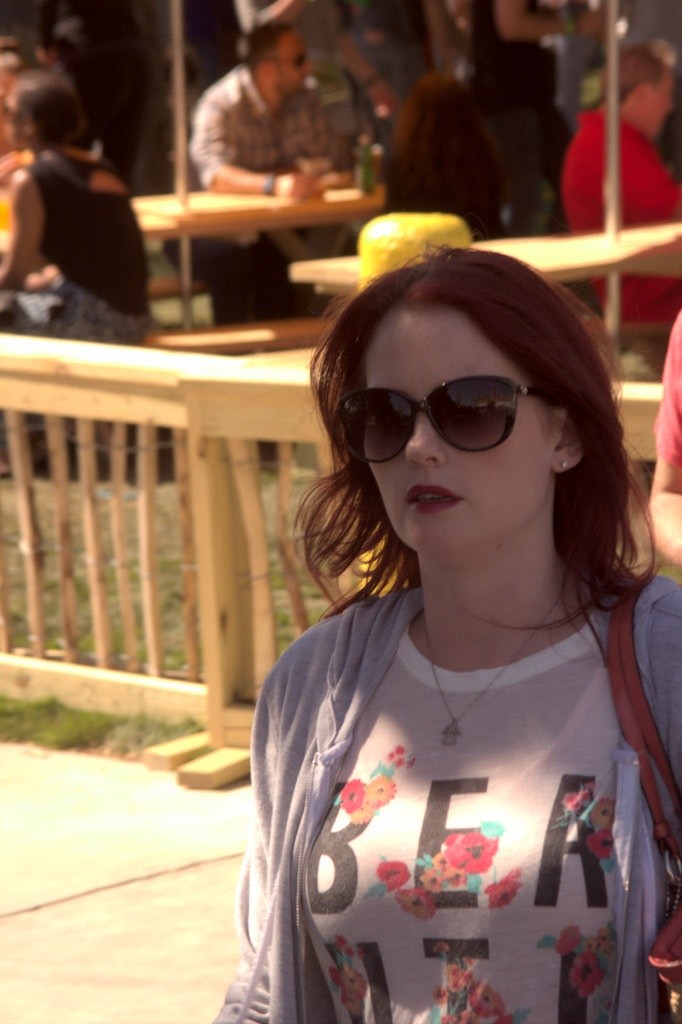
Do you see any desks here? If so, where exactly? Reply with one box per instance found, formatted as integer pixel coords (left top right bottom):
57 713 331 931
288 223 682 354
129 184 387 332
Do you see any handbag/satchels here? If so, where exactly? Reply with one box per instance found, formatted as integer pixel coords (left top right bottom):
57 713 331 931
648 859 682 1024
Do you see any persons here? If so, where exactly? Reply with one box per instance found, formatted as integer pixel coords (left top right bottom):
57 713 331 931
561 40 682 375
445 0 682 237
213 250 682 1023
649 308 682 565
189 0 501 315
0 0 148 344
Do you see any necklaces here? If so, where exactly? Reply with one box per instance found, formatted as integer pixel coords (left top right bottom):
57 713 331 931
424 585 565 746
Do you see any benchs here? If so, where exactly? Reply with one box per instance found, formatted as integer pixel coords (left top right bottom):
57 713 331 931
143 318 340 354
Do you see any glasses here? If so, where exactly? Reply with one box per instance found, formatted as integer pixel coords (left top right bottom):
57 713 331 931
335 376 544 462
271 51 306 69
